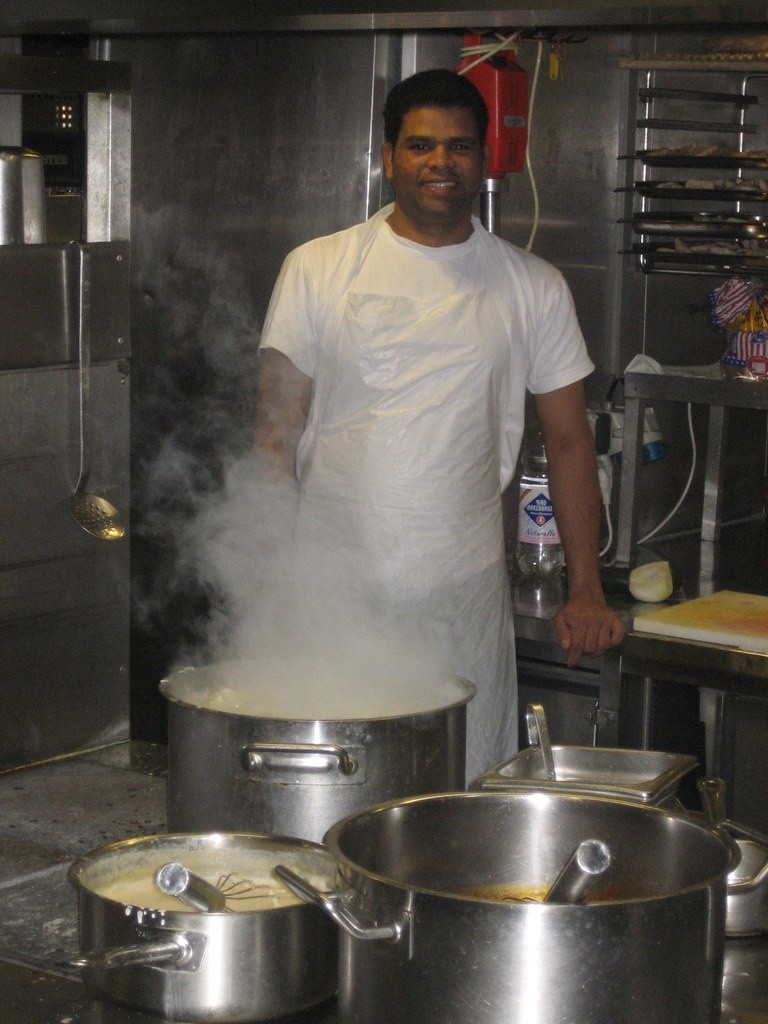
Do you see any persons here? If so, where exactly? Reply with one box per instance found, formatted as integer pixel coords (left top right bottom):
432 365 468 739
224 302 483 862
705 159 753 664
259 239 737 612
251 65 627 796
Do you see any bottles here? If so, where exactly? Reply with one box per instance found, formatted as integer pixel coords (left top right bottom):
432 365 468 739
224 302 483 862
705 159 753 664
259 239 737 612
516 418 564 576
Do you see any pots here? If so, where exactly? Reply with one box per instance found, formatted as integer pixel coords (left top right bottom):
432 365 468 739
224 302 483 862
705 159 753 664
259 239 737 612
67 830 341 1024
158 660 478 844
275 791 768 1024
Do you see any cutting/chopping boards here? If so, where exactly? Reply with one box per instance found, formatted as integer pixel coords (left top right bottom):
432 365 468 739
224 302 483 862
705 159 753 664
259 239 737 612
633 590 768 655
